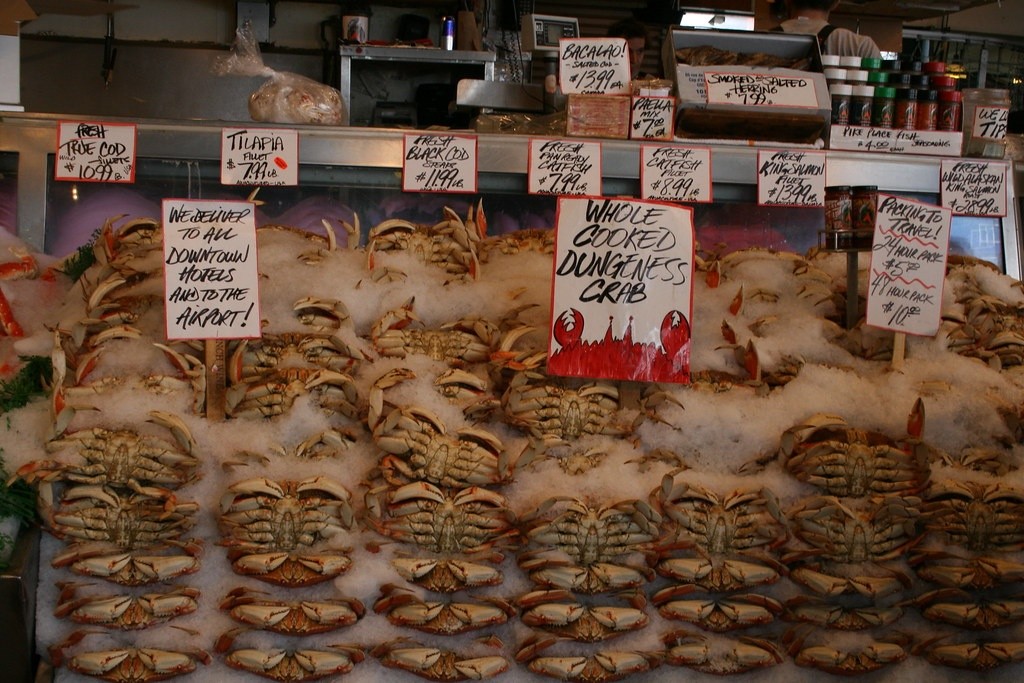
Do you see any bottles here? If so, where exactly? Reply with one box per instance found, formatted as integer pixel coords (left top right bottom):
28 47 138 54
819 54 967 134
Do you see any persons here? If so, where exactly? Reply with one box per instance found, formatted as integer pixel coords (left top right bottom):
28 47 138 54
770 0 882 58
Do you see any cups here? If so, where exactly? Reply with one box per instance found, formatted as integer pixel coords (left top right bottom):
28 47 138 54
341 13 369 45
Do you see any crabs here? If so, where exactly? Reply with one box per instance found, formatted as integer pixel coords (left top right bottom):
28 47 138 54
0 188 1024 683
